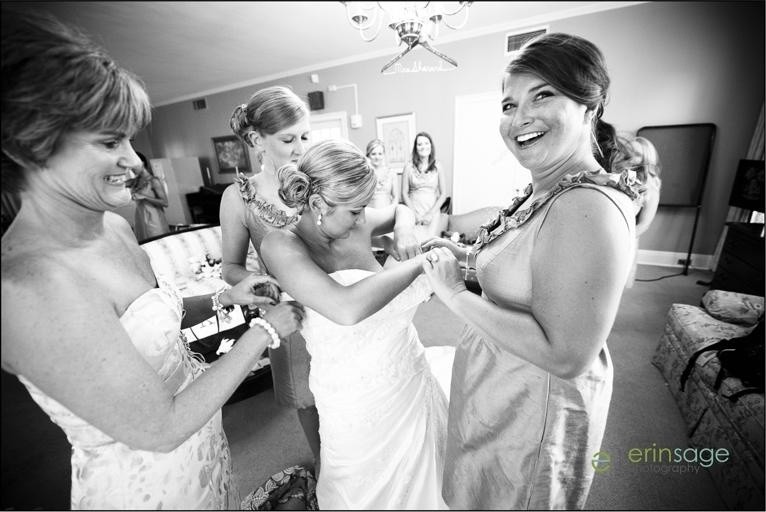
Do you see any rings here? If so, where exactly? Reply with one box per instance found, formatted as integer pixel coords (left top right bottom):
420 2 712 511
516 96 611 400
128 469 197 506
430 252 438 262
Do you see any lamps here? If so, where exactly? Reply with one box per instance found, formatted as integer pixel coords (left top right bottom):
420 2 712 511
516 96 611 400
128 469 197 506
339 0 472 73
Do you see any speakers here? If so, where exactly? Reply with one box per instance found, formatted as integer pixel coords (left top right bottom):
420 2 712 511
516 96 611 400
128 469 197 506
309 92 325 111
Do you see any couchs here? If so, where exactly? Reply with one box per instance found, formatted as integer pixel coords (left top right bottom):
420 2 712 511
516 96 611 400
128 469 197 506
652 286 765 510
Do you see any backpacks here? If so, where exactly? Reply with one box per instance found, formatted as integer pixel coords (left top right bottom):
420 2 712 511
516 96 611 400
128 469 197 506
677 316 764 410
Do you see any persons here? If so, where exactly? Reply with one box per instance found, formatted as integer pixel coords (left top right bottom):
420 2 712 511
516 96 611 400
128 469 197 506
257 136 449 510
421 32 636 512
363 137 400 251
127 150 172 243
0 7 303 510
626 135 664 291
382 130 448 321
222 83 320 482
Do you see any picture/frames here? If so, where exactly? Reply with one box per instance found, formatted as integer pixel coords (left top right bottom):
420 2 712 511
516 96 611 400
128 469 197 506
209 136 253 176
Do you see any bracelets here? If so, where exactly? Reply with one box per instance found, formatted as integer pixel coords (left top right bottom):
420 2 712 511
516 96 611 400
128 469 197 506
210 285 222 313
247 317 280 350
439 280 467 303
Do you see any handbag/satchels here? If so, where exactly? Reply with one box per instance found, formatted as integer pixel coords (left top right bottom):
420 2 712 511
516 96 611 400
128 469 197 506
240 463 318 511
